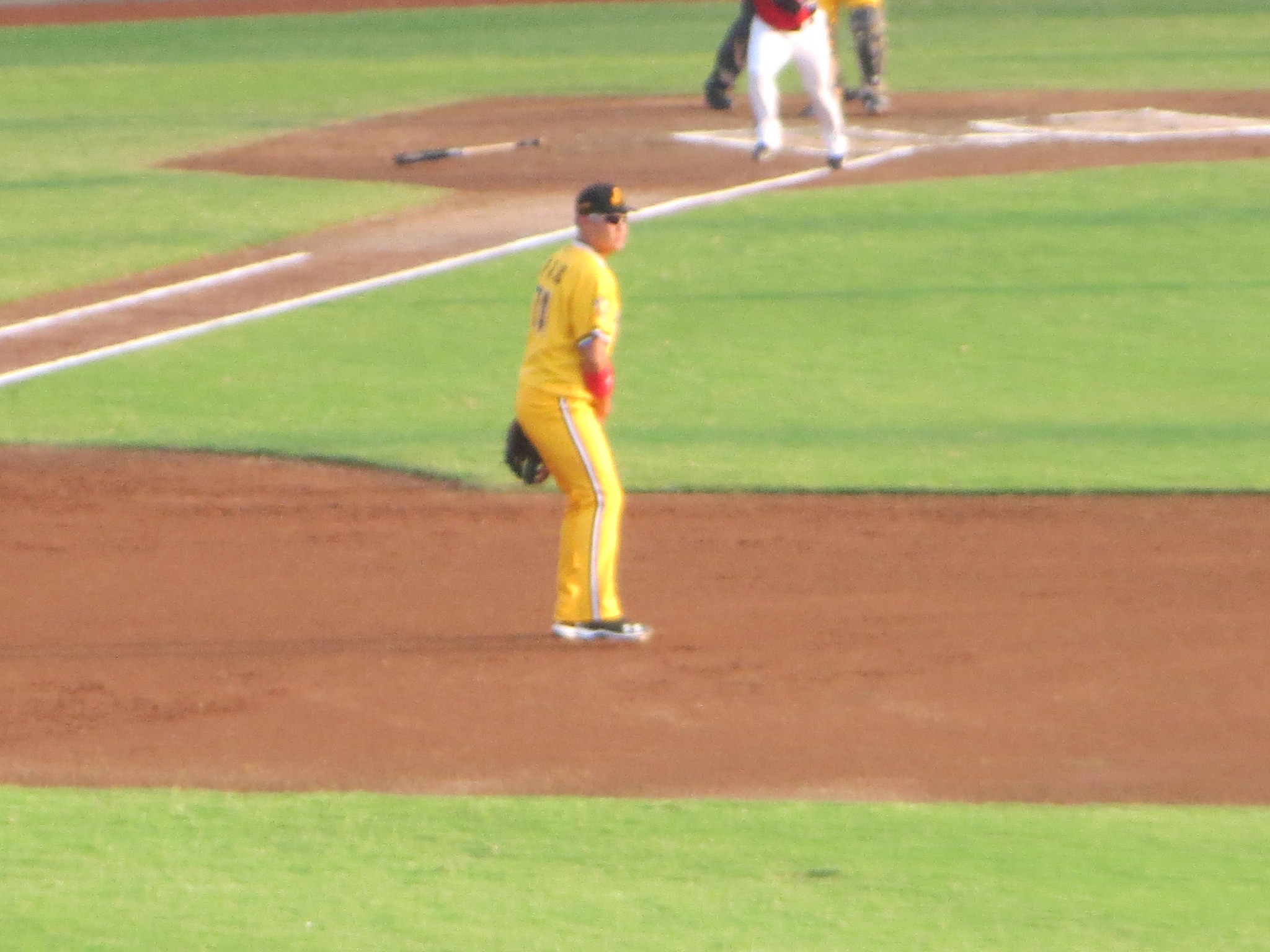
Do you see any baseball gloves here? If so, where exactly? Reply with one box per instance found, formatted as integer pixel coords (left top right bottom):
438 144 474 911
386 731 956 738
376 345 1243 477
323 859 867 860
504 419 550 485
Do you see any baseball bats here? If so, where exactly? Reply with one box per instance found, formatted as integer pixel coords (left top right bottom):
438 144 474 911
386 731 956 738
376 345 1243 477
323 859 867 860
395 138 541 164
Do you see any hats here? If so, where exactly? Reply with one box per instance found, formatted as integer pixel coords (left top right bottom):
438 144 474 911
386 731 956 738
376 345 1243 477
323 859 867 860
575 183 638 214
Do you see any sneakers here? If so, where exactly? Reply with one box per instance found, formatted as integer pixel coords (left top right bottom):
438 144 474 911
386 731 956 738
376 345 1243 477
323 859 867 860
552 618 582 641
578 618 655 642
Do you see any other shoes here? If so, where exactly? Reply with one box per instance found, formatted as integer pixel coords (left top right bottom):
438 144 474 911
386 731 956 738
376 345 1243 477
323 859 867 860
828 153 846 170
755 139 781 160
864 94 890 116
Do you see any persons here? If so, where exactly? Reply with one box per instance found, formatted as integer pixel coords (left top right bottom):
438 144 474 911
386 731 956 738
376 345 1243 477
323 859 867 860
705 0 894 174
516 182 656 642
